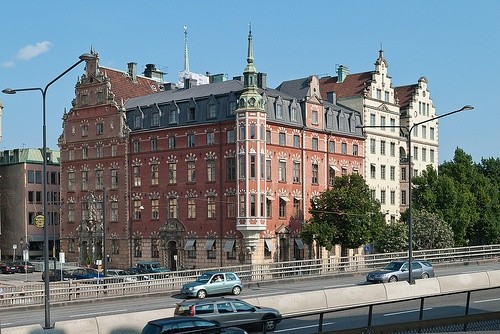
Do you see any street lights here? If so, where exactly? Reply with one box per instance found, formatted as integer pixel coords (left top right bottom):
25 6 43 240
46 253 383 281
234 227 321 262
2 52 95 330
356 105 475 284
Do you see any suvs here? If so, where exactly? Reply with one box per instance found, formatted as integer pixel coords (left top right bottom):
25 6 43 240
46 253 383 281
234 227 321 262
71 268 106 283
179 271 243 300
366 260 435 284
175 297 283 332
140 316 248 334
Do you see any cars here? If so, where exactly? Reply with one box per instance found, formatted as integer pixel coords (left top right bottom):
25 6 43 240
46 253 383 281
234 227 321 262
41 269 72 282
0 260 19 274
101 261 170 281
13 260 35 273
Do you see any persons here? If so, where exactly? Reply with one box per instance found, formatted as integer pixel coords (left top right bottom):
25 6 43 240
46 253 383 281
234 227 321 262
139 270 144 281
215 276 222 282
